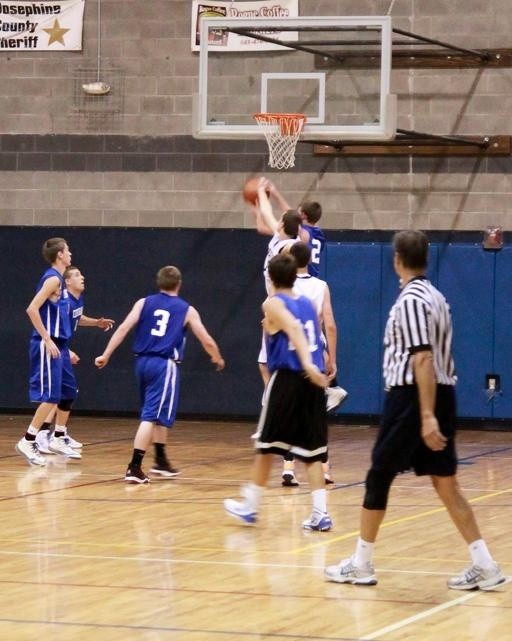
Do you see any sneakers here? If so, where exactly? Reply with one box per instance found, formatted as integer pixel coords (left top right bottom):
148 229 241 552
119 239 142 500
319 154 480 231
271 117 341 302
34 429 56 455
150 464 182 479
324 385 350 414
124 463 150 484
45 426 83 459
318 462 334 485
322 556 378 586
281 459 301 486
221 496 257 524
14 436 48 468
446 562 510 593
302 507 333 531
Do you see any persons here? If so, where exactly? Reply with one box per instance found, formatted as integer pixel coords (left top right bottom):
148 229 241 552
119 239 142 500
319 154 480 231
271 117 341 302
94 266 226 483
14 237 82 468
323 229 508 592
32 266 116 454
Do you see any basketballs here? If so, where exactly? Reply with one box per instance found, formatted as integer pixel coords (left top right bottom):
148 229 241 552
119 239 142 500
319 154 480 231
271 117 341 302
245 177 270 205
